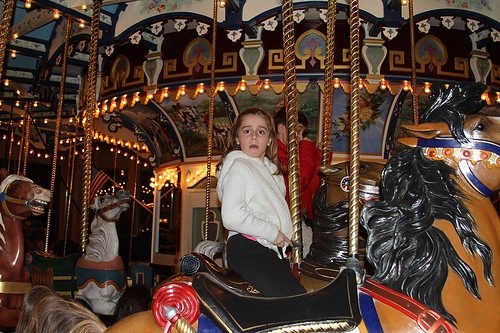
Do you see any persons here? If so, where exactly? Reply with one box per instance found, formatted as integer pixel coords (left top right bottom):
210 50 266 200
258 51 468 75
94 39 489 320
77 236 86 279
213 107 309 298
267 104 327 266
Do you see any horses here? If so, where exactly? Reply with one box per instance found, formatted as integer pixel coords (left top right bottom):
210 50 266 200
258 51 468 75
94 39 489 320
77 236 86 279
15 82 500 333
0 167 128 333
